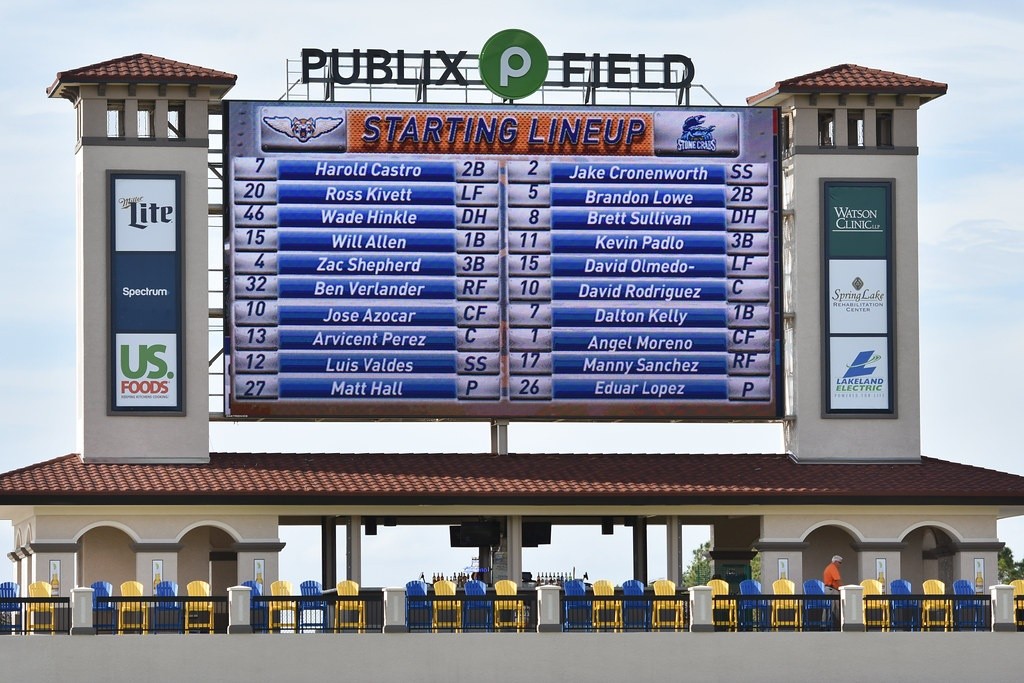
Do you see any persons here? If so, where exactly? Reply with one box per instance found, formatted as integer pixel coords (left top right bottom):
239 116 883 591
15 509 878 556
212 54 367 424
821 555 843 631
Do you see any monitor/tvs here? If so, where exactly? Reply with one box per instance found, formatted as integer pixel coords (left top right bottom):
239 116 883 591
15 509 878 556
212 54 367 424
449 521 501 547
522 521 552 547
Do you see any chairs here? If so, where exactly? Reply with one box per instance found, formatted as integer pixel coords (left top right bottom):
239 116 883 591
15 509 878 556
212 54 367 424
890 579 920 631
563 579 592 632
771 578 803 632
185 580 214 635
1009 579 1024 625
802 578 834 631
432 580 462 633
90 580 117 635
653 579 684 632
592 580 623 633
405 580 433 633
153 581 184 635
953 580 985 632
859 578 890 632
738 579 773 632
462 579 493 632
0 581 24 635
269 580 297 634
25 581 56 635
494 579 524 633
118 580 149 635
333 579 367 634
298 580 328 633
707 579 738 632
921 579 949 632
621 580 652 632
239 580 268 635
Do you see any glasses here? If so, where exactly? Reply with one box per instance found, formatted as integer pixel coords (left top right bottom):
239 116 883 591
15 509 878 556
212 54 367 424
835 561 842 564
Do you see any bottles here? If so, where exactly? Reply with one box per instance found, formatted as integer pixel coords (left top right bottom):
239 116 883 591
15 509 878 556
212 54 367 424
536 571 573 588
153 567 161 597
255 567 264 596
436 572 469 588
50 569 60 596
780 561 787 579
975 562 983 595
877 562 885 594
420 572 425 583
433 573 436 583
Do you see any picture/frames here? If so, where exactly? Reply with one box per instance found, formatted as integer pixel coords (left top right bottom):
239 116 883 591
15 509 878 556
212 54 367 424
777 558 790 580
875 557 887 594
974 557 985 594
253 558 265 596
151 559 163 596
48 559 61 598
